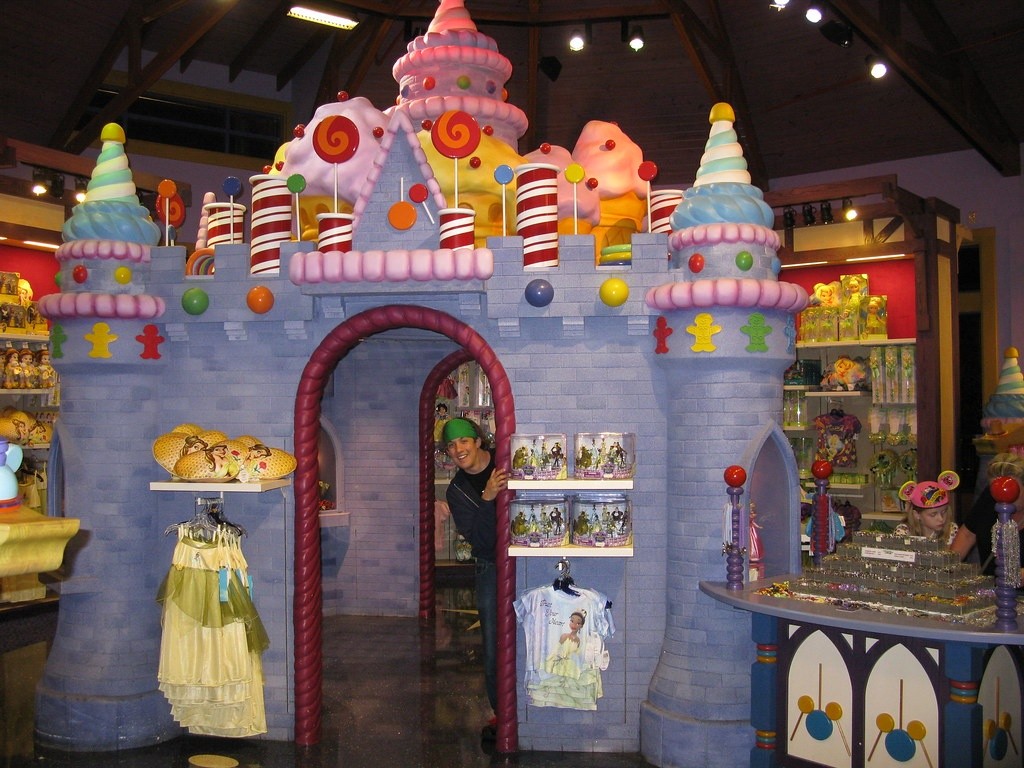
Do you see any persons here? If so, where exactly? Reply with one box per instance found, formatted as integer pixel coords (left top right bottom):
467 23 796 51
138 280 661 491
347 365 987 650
572 503 631 537
512 437 567 474
749 499 765 562
544 609 588 679
512 504 564 536
892 451 1024 576
576 438 628 472
442 416 511 741
3 347 58 388
433 403 450 443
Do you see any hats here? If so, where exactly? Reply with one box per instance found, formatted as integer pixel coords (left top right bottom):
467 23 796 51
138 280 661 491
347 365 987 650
898 471 960 508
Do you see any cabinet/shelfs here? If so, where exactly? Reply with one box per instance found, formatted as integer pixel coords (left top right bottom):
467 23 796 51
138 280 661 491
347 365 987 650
508 476 634 557
783 337 916 552
0 269 61 449
434 479 483 651
698 572 1024 768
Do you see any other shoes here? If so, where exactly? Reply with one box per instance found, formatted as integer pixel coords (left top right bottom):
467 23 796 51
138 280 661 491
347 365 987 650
482 716 496 740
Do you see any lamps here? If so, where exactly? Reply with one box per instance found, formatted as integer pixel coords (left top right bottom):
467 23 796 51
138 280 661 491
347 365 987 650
819 19 854 49
285 1 359 30
842 198 857 220
802 204 817 226
820 201 834 225
32 166 47 196
629 25 644 52
74 175 87 202
570 28 584 51
865 55 887 78
783 205 797 229
806 1 826 24
50 169 64 200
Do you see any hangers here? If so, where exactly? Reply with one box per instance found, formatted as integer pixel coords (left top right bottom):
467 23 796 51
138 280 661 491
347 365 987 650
17 456 44 483
163 497 248 539
553 559 612 609
